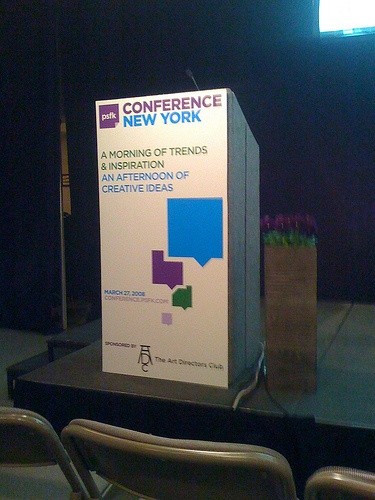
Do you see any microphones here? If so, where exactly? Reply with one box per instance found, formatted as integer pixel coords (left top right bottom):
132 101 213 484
185 69 200 91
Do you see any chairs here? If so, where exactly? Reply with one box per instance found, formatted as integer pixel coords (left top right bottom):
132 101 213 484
302 464 375 500
0 400 112 500
60 413 301 500
